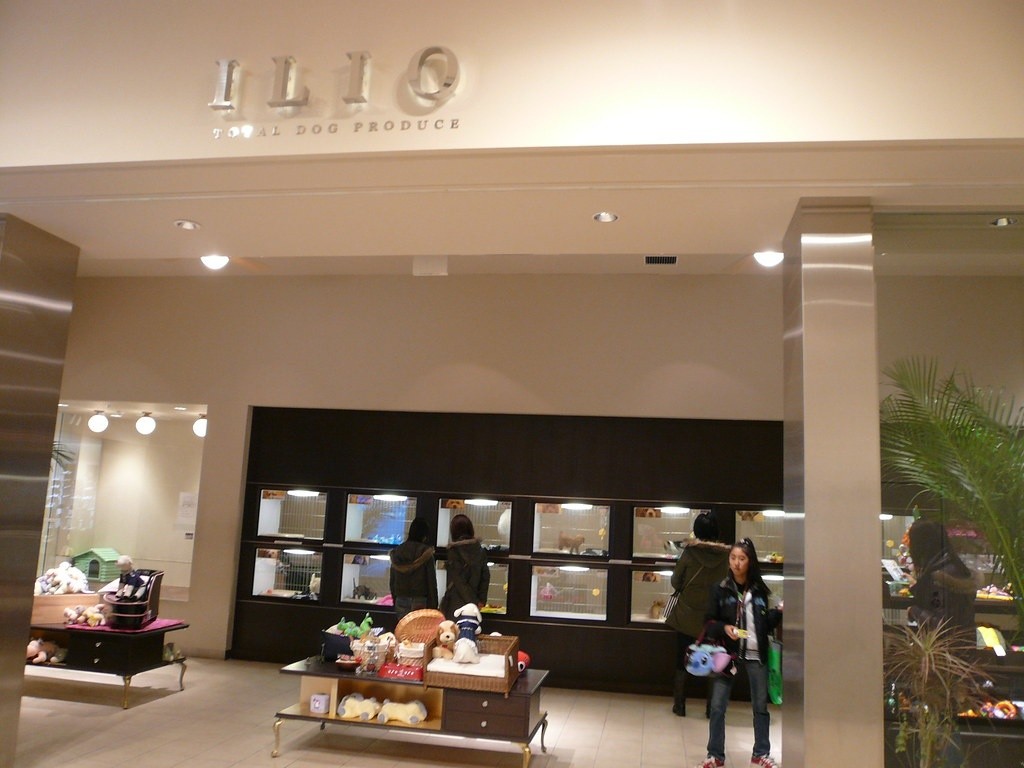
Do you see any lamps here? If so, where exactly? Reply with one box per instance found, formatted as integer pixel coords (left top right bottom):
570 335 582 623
191 413 207 437
87 410 109 433
135 411 156 435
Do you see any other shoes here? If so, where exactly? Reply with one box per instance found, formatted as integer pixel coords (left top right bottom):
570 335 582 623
672 704 685 716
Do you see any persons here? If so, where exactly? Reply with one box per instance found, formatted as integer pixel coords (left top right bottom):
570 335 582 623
697 538 781 768
437 514 490 623
387 514 438 621
904 516 978 768
664 514 730 719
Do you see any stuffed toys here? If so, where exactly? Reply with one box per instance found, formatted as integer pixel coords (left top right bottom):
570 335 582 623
337 693 381 721
34 561 87 595
24 637 68 663
63 603 107 627
454 602 483 663
337 615 373 638
115 556 144 600
432 619 458 659
375 699 427 724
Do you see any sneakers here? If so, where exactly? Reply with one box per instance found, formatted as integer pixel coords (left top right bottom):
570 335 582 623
750 753 778 768
698 754 724 768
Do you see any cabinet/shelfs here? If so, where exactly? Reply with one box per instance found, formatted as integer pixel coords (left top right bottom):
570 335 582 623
234 406 1024 702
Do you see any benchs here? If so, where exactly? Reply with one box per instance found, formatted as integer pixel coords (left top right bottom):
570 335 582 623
420 631 521 698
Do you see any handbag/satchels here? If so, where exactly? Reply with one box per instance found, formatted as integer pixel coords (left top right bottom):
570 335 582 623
768 640 784 704
684 620 732 677
663 591 680 618
439 595 448 613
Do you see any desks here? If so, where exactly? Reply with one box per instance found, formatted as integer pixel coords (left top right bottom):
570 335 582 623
26 614 196 711
270 655 555 768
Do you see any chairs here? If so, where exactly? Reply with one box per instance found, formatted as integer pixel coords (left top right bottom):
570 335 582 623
103 567 164 630
